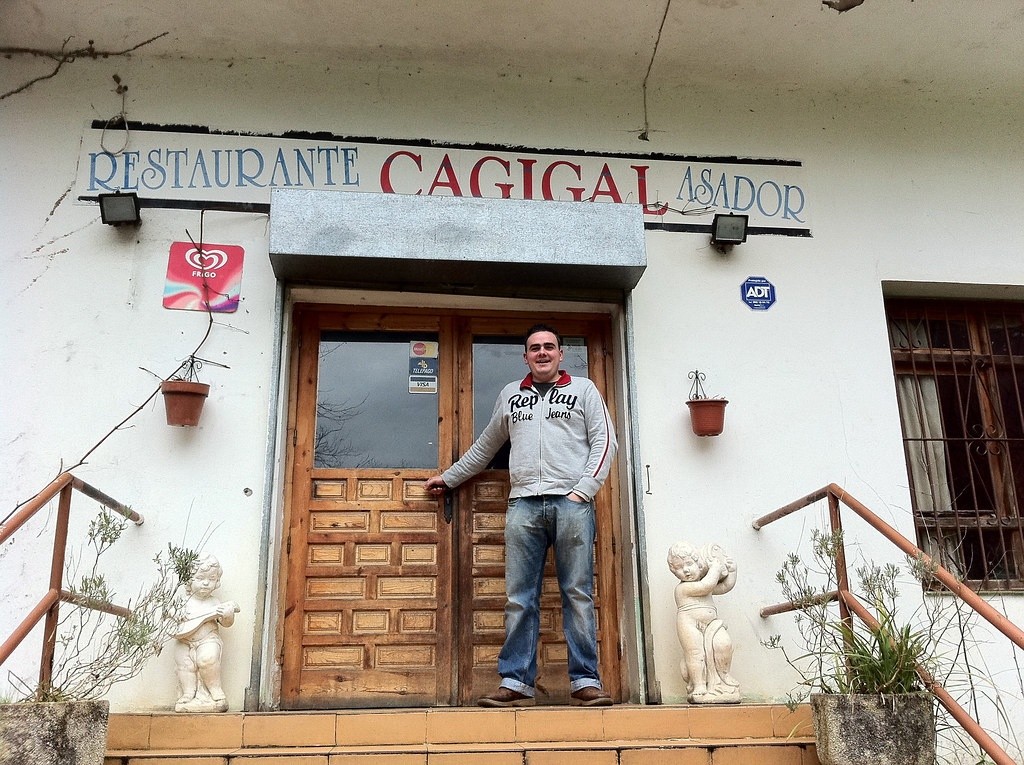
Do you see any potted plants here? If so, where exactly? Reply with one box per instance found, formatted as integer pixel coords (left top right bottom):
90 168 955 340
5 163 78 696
763 525 938 765
0 497 225 765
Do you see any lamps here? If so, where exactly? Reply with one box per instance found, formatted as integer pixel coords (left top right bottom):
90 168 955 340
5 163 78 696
98 189 142 227
710 211 749 254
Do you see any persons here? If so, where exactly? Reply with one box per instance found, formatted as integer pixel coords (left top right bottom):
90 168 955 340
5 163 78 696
166 551 235 704
423 323 619 707
666 540 737 696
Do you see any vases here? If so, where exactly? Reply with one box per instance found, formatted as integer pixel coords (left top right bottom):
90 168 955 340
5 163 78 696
687 400 729 437
160 380 210 428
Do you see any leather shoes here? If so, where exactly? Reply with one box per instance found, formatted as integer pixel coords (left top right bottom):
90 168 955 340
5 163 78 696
477 685 536 707
569 686 613 706
